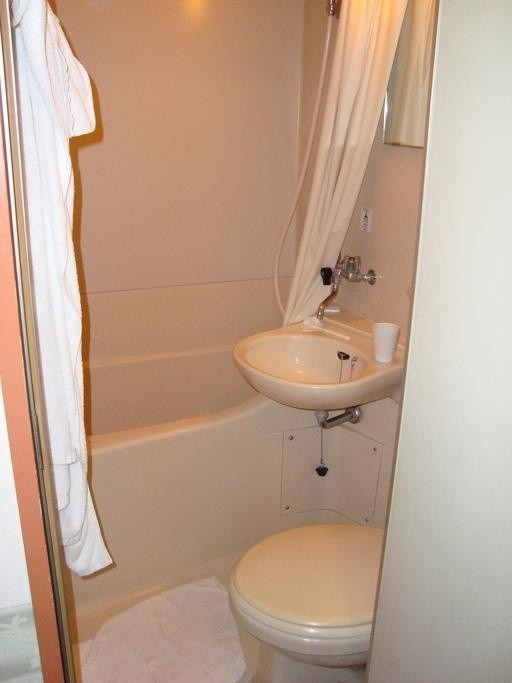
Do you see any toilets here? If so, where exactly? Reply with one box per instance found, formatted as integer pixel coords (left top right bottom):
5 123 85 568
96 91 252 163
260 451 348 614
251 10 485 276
230 519 383 681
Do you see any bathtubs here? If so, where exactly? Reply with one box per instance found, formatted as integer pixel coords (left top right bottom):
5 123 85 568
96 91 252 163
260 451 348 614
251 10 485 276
66 343 271 643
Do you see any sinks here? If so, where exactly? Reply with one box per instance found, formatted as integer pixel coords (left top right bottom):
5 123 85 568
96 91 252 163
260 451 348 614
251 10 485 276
233 310 407 412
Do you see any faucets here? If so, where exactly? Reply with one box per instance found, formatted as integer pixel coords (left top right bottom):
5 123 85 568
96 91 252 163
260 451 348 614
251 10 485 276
316 251 369 320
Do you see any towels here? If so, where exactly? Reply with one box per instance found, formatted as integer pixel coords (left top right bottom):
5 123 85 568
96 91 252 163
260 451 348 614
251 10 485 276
72 573 246 681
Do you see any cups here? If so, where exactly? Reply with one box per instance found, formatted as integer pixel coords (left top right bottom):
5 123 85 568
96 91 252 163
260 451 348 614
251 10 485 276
372 322 400 363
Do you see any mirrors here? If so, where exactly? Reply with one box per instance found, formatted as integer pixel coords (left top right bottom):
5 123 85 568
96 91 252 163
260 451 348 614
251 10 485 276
382 0 438 149
